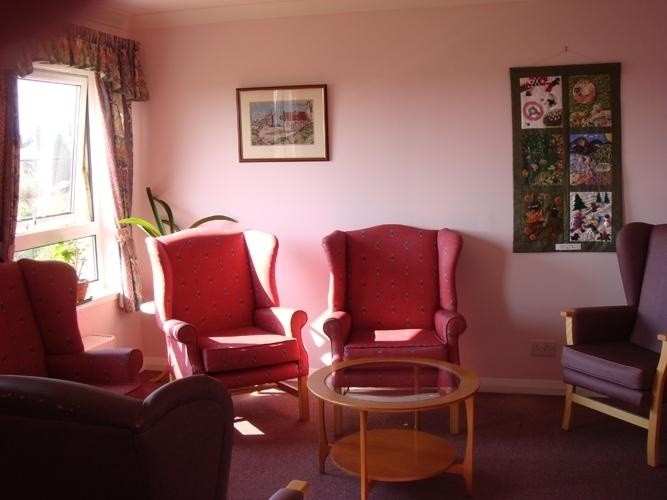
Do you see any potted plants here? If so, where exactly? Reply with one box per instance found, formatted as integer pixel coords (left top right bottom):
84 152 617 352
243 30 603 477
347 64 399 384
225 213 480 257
50 237 89 304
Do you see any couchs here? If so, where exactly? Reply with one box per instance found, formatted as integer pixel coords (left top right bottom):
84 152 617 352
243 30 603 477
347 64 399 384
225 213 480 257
0 374 310 499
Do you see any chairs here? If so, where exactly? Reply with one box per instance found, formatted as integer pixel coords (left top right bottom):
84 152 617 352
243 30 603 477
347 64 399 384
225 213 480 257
322 222 469 394
145 227 309 422
558 221 666 467
0 258 145 396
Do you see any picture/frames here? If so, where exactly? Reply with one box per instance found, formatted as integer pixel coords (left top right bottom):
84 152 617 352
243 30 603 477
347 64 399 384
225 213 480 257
235 83 330 162
